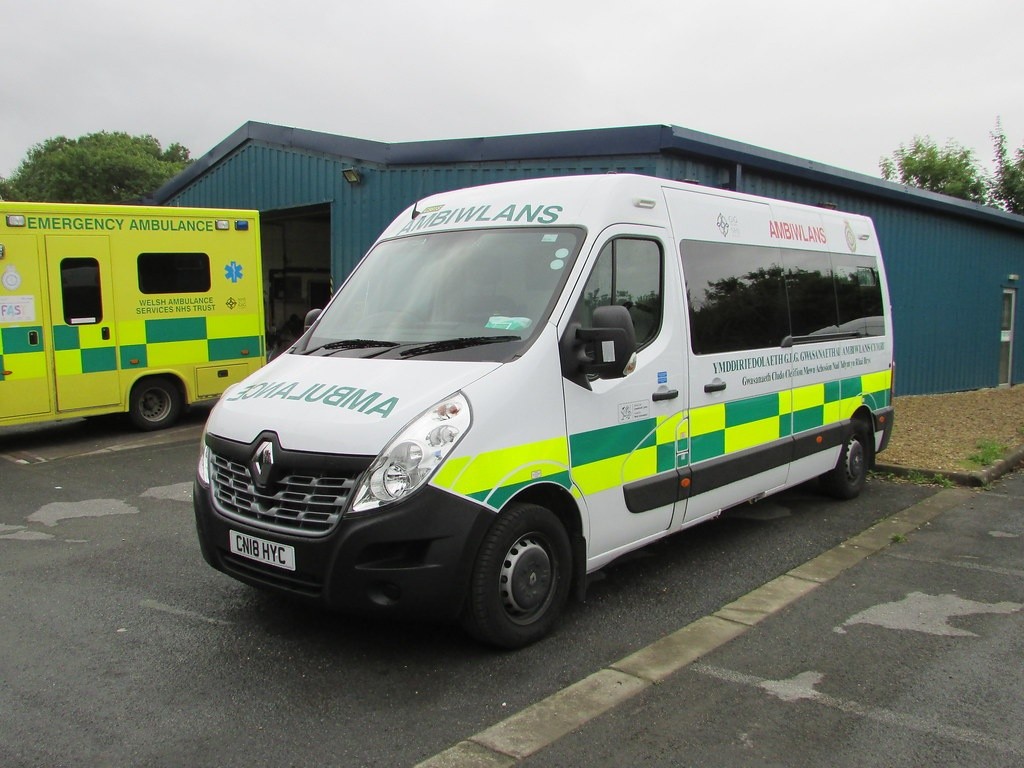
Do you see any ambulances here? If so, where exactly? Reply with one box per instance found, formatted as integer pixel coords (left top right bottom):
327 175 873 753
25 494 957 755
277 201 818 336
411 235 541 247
195 173 894 650
0 202 267 431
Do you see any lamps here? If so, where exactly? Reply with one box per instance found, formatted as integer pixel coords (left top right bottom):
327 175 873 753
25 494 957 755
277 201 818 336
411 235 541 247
341 167 364 184
